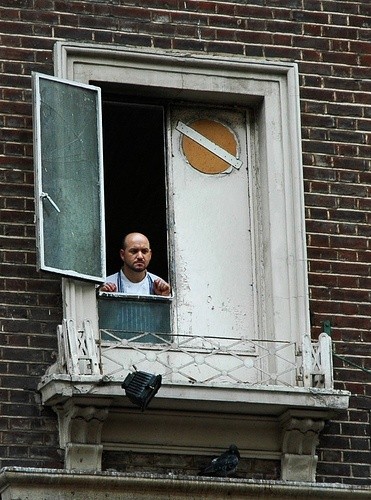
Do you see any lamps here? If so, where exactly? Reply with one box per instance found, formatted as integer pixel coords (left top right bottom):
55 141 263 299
120 370 163 411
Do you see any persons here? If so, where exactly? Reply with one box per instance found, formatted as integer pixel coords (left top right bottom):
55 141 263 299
95 231 171 296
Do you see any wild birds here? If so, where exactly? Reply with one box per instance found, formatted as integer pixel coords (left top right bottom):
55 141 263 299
195 443 242 478
